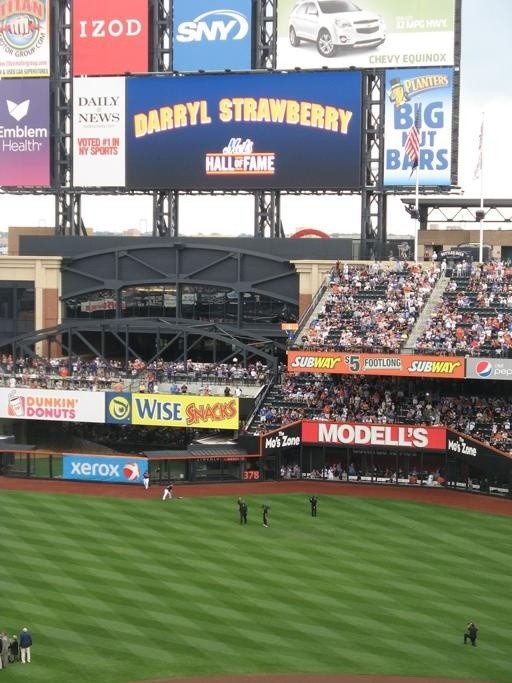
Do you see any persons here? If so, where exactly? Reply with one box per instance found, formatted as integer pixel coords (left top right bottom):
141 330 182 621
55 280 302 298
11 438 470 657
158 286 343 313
161 483 174 500
443 257 512 359
238 497 248 524
480 477 489 487
298 258 447 354
463 622 479 647
143 471 152 490
281 462 446 487
465 476 473 487
261 502 270 527
308 495 318 516
20 626 34 664
0 630 19 669
253 361 511 455
370 249 438 262
0 349 267 404
62 421 208 451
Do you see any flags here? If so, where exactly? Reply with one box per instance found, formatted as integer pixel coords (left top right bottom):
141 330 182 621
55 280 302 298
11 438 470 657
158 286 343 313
405 103 420 177
471 116 484 180
